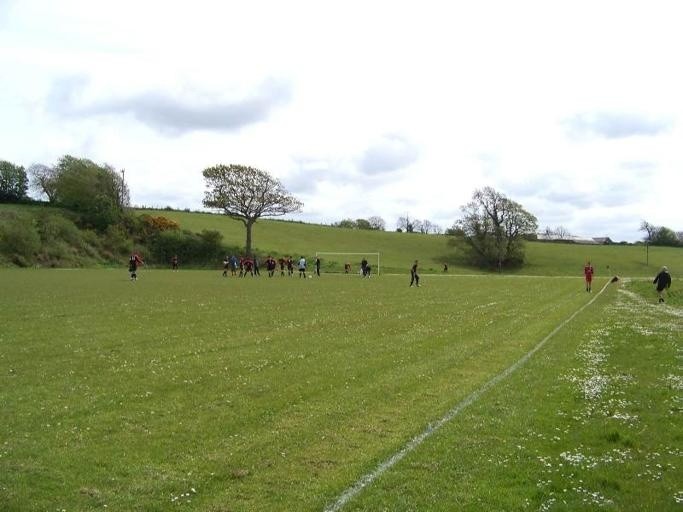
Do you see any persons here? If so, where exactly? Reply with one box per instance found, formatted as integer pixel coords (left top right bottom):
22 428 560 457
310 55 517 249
221 254 454 287
651 264 671 303
585 262 593 292
170 253 176 267
127 250 143 281
